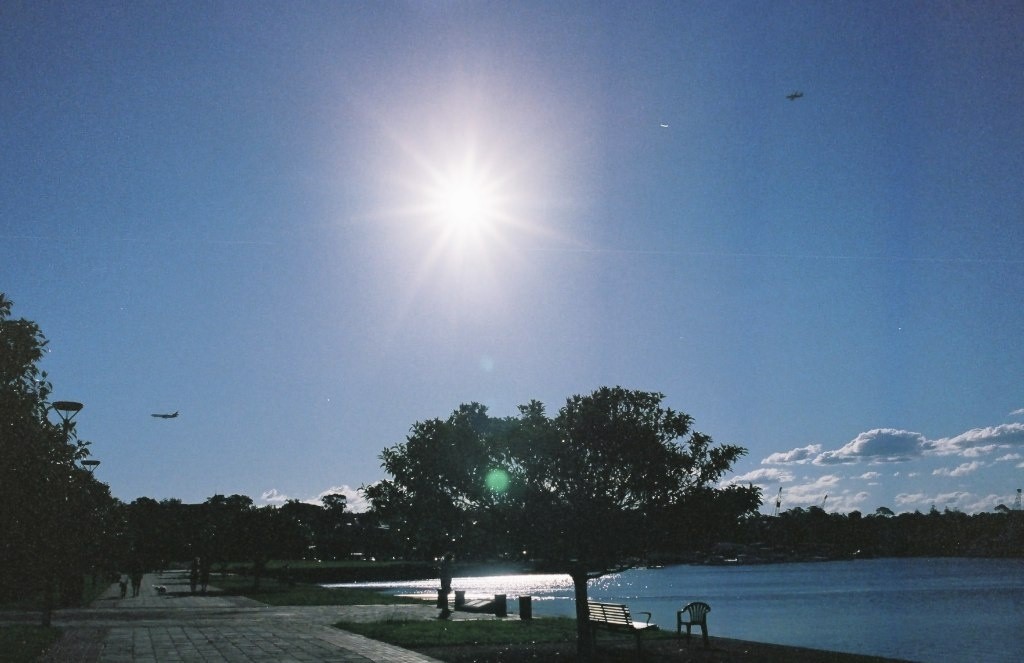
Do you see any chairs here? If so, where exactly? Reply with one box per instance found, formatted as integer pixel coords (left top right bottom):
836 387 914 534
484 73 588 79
678 602 711 644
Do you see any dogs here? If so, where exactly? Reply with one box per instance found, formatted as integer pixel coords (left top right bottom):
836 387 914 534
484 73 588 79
119 581 127 599
154 586 167 595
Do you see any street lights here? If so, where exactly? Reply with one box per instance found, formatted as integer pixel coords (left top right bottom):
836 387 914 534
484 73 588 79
77 460 100 600
40 401 83 632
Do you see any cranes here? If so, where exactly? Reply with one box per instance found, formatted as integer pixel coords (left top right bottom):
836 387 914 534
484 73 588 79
774 487 783 515
821 494 828 509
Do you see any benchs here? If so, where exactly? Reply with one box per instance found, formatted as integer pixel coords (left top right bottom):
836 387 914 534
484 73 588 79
585 601 660 658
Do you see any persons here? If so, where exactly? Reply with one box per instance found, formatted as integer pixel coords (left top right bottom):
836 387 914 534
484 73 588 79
119 554 164 598
189 555 212 594
